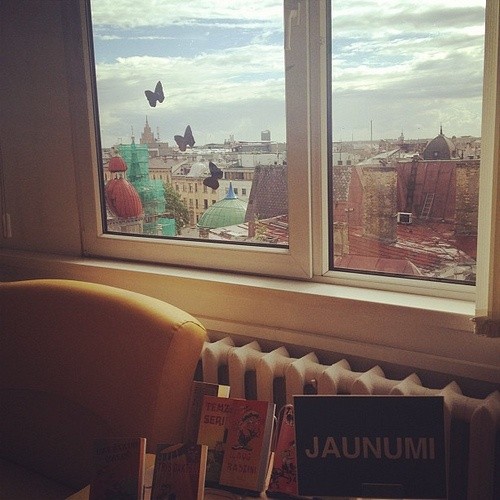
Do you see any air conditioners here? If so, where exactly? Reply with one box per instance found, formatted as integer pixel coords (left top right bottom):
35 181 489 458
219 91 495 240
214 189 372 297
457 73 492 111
397 211 413 225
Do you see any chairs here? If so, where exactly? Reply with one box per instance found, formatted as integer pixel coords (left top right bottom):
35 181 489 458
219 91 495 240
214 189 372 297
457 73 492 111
0 274 209 500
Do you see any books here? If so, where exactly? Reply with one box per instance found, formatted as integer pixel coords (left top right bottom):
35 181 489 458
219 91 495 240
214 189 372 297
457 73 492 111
88 380 451 500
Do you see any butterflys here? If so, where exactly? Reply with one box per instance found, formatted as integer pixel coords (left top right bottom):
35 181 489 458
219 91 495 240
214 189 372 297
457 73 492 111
174 125 196 152
145 80 165 107
202 162 223 191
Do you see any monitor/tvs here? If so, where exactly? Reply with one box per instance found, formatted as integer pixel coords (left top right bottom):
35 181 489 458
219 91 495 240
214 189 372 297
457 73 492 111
292 394 448 499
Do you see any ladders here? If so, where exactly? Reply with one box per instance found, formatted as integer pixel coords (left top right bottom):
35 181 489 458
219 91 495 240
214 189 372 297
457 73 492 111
418 192 437 219
